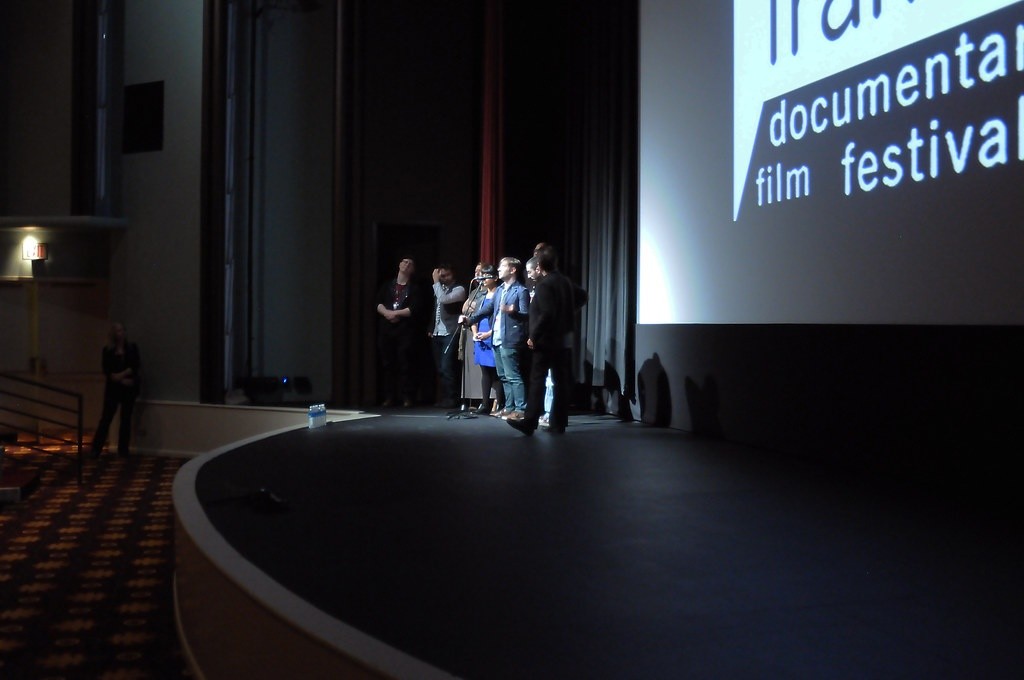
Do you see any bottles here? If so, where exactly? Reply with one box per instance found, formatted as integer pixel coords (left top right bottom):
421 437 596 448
307 404 327 428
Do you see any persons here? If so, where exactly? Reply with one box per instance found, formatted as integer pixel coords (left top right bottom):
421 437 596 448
81 323 140 460
374 242 574 437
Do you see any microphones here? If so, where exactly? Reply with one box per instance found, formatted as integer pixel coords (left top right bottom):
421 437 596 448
474 275 494 280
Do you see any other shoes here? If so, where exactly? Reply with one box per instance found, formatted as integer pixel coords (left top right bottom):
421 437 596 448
403 399 412 407
506 418 535 436
383 399 393 406
489 402 503 415
476 407 491 415
433 396 460 409
538 412 550 426
494 406 515 418
501 410 524 420
542 425 566 433
90 446 100 458
117 447 130 458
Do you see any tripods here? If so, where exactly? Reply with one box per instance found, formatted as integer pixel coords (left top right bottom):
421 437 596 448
445 280 498 420
